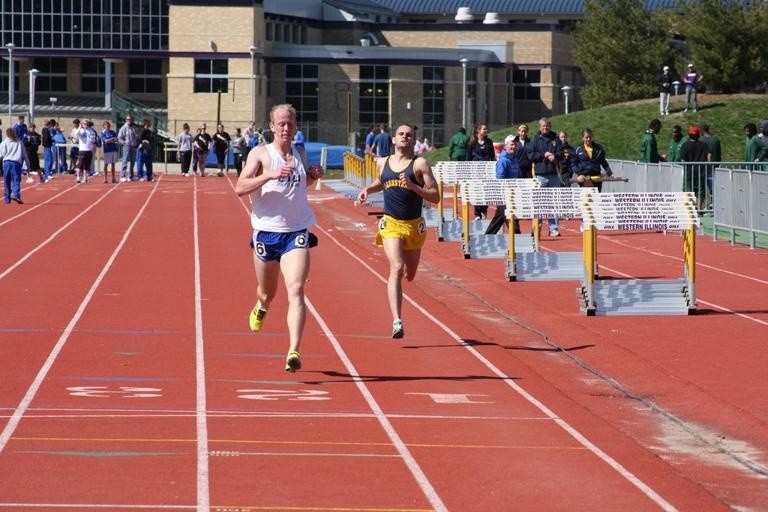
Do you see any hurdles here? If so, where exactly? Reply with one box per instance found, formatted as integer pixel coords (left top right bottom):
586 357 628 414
575 190 698 310
163 142 195 176
422 161 497 242
502 187 599 282
55 143 103 184
459 178 541 260
581 221 700 315
320 149 385 206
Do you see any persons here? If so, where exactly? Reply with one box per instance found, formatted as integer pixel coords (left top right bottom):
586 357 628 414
12 115 68 183
292 127 305 151
681 60 704 116
352 120 441 340
448 117 612 236
68 114 157 184
365 122 436 155
234 106 323 372
743 118 768 171
1 128 31 204
655 64 673 120
177 121 268 178
640 120 720 210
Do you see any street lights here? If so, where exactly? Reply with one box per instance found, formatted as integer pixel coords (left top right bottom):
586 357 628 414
28 68 40 122
247 44 258 121
459 56 470 131
560 84 571 114
5 43 16 126
672 79 681 95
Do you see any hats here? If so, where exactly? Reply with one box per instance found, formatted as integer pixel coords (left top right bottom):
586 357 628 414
504 135 519 143
689 128 700 135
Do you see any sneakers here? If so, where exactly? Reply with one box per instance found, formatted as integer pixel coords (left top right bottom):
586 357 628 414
249 300 268 331
77 177 87 183
392 322 403 338
181 173 225 177
104 174 155 183
285 351 302 372
475 212 486 220
10 193 23 204
550 229 559 237
25 171 54 183
